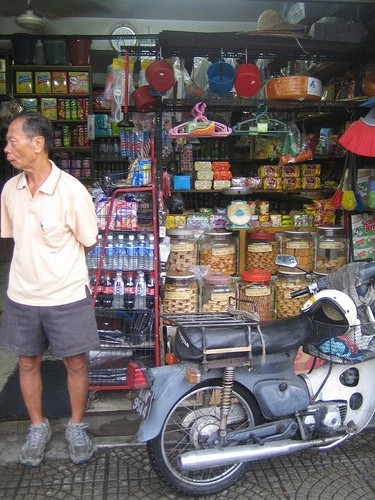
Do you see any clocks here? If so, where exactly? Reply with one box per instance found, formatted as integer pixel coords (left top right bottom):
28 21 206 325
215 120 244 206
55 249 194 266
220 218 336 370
108 24 137 57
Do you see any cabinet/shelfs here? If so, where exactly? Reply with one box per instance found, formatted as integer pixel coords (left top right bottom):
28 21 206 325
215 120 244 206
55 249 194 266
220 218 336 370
1 36 374 372
155 90 375 377
84 159 154 394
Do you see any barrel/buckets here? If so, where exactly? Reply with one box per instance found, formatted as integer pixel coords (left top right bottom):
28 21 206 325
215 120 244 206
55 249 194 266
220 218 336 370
237 65 261 96
145 60 176 93
206 62 237 95
130 85 156 108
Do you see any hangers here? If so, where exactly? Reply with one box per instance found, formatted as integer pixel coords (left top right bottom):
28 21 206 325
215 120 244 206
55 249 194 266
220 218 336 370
235 100 290 133
168 102 232 137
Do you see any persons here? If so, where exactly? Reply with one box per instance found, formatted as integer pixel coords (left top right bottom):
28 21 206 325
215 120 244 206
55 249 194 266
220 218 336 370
1 111 100 467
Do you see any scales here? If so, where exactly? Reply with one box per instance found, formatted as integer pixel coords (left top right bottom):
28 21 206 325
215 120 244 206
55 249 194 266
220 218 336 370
220 186 255 229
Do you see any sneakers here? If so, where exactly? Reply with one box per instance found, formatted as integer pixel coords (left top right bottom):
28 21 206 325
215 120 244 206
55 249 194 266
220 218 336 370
64 418 94 464
18 418 52 466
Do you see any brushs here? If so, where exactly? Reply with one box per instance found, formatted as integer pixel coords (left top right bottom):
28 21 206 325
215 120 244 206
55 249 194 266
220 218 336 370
331 152 365 213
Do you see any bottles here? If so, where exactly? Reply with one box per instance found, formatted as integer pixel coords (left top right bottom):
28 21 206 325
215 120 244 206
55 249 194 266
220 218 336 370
276 271 311 317
97 138 119 159
84 233 156 308
63 126 90 148
201 276 236 312
201 231 237 276
247 229 279 274
167 229 198 276
317 226 351 274
160 277 198 325
58 98 89 120
283 230 315 272
240 270 274 316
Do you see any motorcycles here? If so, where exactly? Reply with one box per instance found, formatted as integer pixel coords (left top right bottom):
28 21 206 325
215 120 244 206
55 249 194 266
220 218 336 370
126 254 375 497
